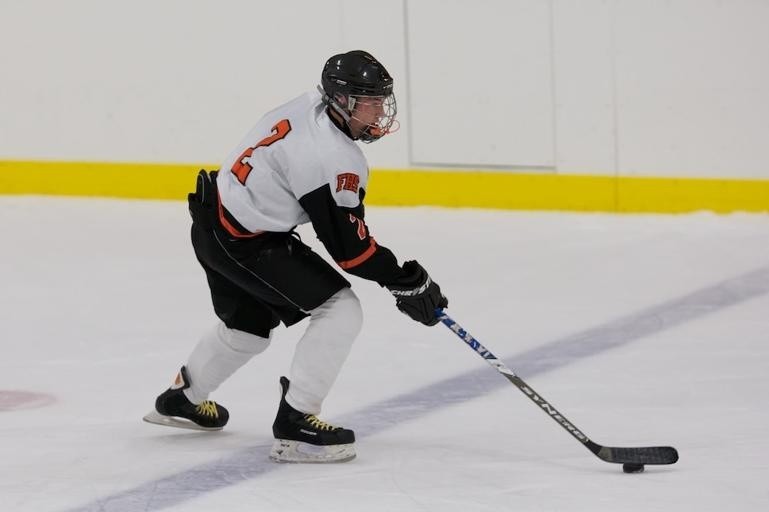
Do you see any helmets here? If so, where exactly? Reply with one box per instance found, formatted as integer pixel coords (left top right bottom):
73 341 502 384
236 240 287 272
320 50 396 120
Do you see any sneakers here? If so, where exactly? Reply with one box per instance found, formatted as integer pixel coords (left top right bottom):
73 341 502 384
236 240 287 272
154 365 230 428
271 375 356 445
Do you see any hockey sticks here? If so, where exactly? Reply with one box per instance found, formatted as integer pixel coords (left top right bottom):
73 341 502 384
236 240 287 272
437 311 678 466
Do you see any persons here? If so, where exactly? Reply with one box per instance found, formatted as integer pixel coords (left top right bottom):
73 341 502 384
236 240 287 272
154 50 445 447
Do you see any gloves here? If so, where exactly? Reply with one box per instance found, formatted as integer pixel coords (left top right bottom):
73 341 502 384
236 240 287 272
382 260 450 327
